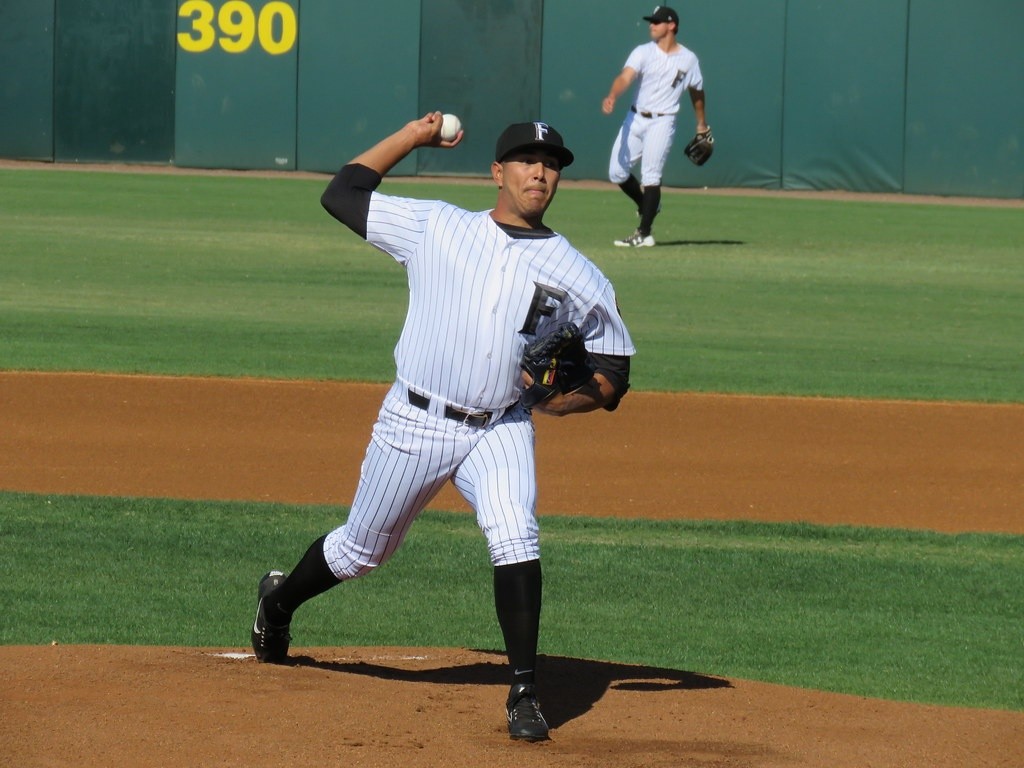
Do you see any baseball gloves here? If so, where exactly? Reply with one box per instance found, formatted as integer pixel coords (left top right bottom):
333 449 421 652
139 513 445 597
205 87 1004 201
518 320 596 410
683 124 715 166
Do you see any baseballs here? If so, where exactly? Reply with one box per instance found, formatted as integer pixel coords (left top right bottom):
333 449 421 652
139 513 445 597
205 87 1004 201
438 112 463 141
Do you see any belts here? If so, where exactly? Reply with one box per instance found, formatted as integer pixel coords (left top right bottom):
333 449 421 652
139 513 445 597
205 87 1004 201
631 106 667 119
407 389 522 429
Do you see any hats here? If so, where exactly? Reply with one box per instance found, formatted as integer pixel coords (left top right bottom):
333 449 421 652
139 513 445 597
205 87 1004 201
642 5 679 23
495 121 574 167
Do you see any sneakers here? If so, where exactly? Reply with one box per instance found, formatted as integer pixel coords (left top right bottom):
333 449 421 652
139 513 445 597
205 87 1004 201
613 227 656 247
506 680 549 740
251 570 293 663
636 201 661 218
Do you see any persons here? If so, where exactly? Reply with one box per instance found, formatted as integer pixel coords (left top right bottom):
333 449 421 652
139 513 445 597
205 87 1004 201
250 111 638 742
601 6 714 248
